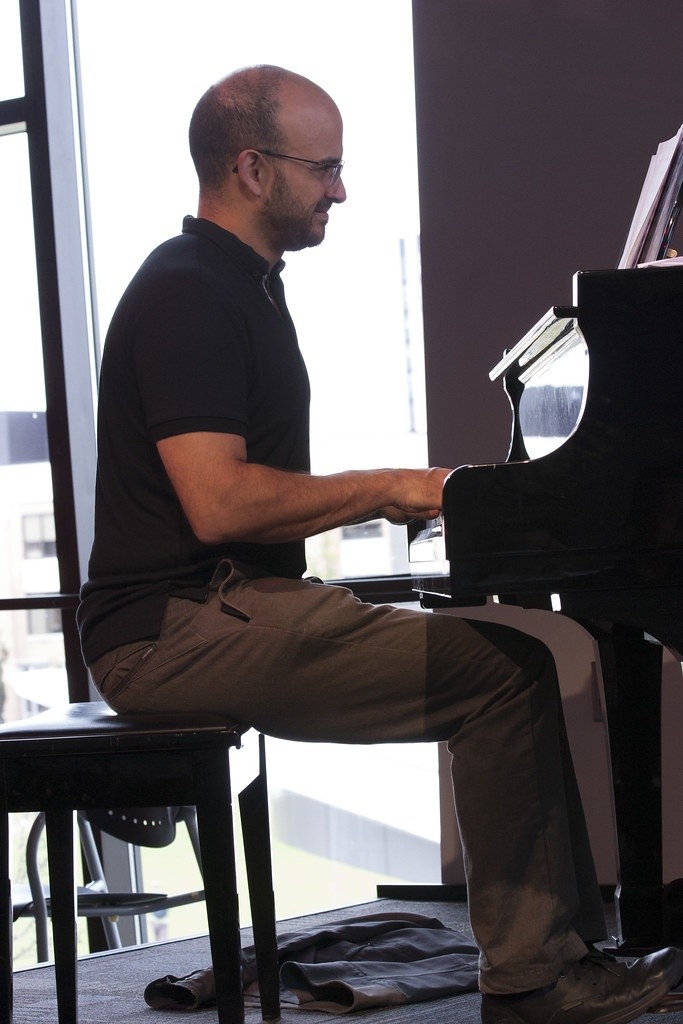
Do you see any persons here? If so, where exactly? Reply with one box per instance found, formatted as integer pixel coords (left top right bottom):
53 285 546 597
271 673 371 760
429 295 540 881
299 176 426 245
76 66 683 1024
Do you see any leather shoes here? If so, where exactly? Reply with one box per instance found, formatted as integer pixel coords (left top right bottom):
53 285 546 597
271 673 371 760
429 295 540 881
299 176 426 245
481 945 681 1024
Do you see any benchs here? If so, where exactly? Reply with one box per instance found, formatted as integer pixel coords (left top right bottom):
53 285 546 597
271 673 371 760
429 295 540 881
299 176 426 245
0 700 283 1024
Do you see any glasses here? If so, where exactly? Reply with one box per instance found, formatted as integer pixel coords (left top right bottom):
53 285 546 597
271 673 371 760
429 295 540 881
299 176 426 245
231 149 344 189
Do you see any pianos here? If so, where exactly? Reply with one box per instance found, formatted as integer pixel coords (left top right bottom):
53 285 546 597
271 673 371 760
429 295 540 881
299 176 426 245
400 267 683 961
